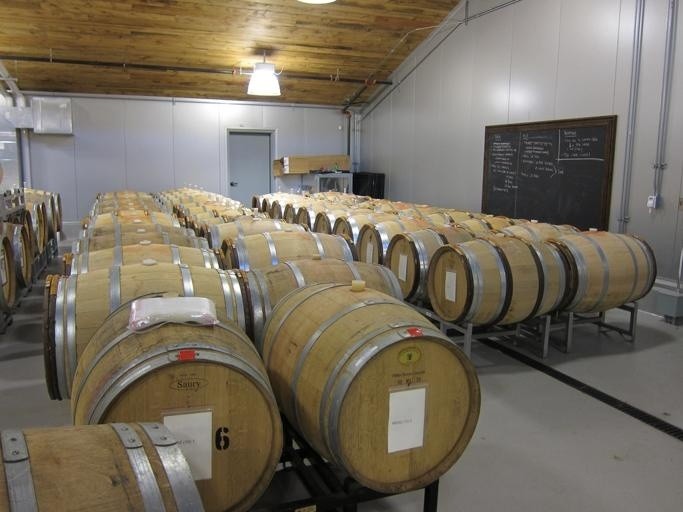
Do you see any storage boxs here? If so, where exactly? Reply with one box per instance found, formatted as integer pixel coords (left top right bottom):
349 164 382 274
271 155 351 178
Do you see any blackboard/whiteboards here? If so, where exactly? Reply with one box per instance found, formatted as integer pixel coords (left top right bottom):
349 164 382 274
480 114 618 230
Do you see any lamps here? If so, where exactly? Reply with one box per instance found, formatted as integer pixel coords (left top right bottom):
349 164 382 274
246 48 281 97
